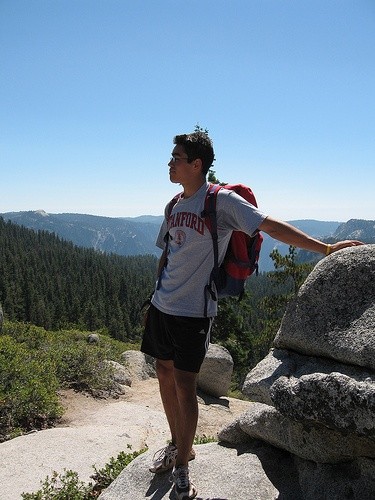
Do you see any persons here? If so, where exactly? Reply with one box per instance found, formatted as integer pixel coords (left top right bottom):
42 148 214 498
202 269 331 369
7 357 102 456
139 134 365 500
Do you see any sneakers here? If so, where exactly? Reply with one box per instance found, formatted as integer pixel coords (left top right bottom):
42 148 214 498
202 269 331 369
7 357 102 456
148 441 197 500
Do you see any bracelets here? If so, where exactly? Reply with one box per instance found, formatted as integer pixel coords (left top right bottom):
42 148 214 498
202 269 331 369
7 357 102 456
326 244 331 256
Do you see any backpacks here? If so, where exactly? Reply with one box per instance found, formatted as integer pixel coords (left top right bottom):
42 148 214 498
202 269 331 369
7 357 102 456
135 183 263 322
